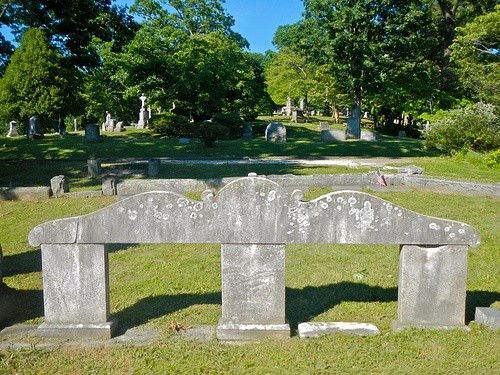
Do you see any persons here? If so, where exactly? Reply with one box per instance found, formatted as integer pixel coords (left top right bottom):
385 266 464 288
333 108 339 124
104 111 111 131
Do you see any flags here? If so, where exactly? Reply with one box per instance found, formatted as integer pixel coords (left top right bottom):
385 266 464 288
377 165 386 186
